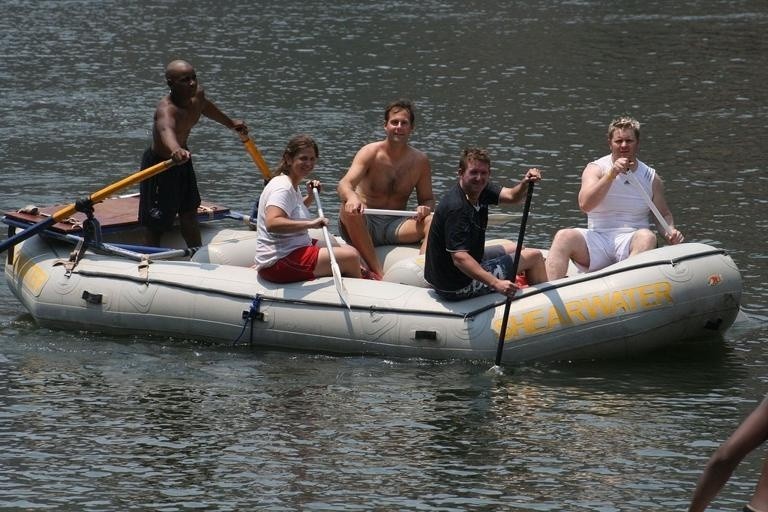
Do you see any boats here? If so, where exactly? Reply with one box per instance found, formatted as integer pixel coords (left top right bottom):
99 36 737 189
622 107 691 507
0 190 743 361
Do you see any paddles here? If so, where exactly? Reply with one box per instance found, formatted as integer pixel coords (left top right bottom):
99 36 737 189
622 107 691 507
360 207 533 229
625 165 753 323
311 182 350 308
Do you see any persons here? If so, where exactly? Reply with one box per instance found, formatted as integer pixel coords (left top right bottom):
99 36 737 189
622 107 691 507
336 98 435 279
137 59 249 247
423 147 549 300
254 134 362 284
543 116 685 282
